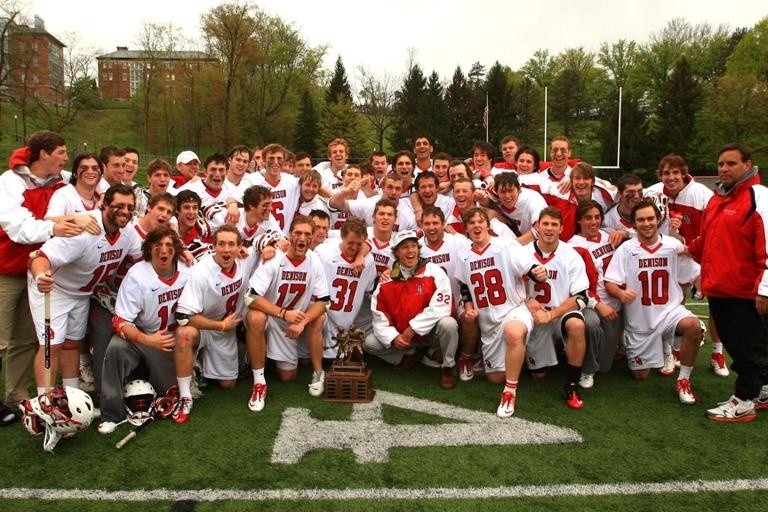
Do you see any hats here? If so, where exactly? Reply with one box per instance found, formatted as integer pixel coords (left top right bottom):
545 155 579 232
176 150 201 165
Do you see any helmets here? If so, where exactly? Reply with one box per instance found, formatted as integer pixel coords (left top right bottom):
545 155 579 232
698 319 707 347
390 229 418 252
19 385 92 452
122 379 157 428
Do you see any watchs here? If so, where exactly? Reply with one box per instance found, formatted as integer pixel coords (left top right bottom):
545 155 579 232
227 201 236 206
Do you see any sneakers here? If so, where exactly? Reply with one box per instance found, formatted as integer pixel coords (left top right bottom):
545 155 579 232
154 384 181 416
308 370 325 397
710 352 730 378
753 385 768 409
390 347 422 370
579 374 593 388
660 353 675 375
173 396 195 422
674 350 682 367
565 382 584 410
496 389 517 419
706 395 756 422
441 367 456 388
248 382 267 412
98 421 119 434
675 378 696 403
458 362 473 381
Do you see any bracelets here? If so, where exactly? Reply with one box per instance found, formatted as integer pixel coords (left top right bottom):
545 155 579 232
547 311 552 322
283 309 287 321
35 273 46 280
134 332 141 341
530 227 539 241
221 321 226 331
277 308 284 318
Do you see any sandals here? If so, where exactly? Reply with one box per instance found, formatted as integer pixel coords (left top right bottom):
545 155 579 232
1 404 16 423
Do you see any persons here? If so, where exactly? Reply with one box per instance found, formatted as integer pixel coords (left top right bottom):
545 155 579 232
163 224 249 424
442 179 507 239
78 333 99 392
438 140 496 202
639 154 732 378
360 228 460 390
313 215 377 361
392 150 417 199
342 321 367 365
98 144 128 197
400 169 464 238
25 183 196 396
561 199 632 390
91 191 178 400
166 150 202 199
330 166 362 196
213 184 289 271
138 159 172 223
94 225 192 436
508 147 542 191
451 207 550 419
367 151 389 183
447 160 473 194
171 190 215 266
323 325 349 364
328 173 416 235
431 151 451 186
462 134 584 194
282 149 296 176
412 204 481 383
120 146 140 191
605 200 706 406
488 168 549 244
278 209 373 275
599 174 686 374
250 145 267 174
238 216 332 412
563 163 617 235
43 153 103 223
246 142 333 235
674 142 768 424
412 134 437 176
182 154 242 232
311 137 349 197
222 146 254 201
515 207 591 408
358 162 377 187
0 129 83 422
357 198 401 286
292 153 313 178
296 169 331 218
